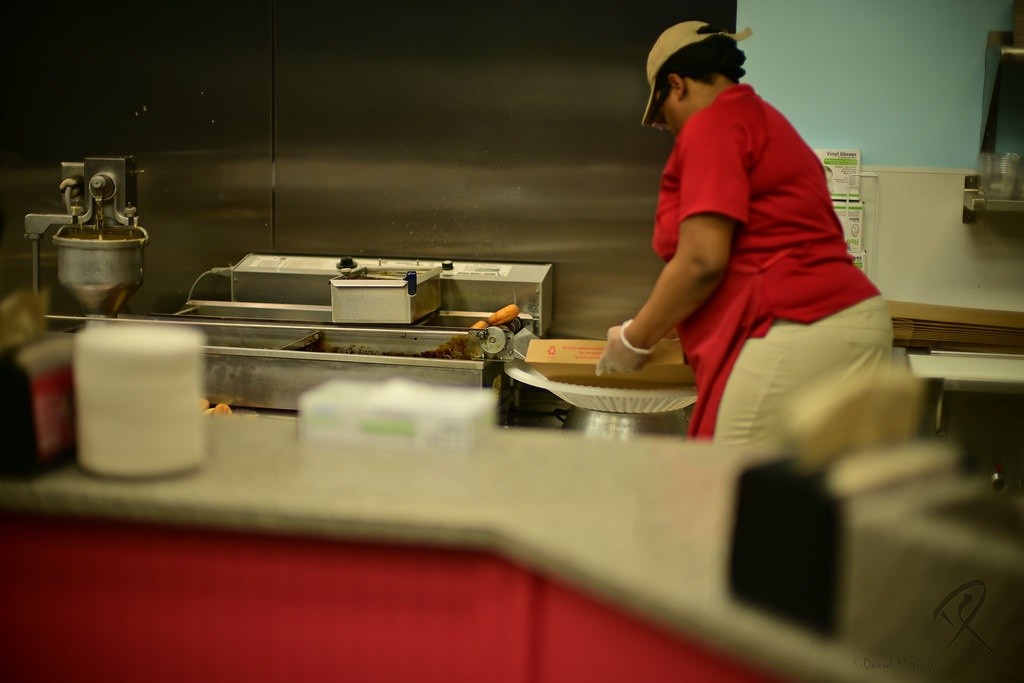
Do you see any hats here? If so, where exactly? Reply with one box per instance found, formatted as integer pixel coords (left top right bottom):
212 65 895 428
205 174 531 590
642 21 753 129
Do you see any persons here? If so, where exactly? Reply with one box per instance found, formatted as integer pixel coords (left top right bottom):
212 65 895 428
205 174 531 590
592 19 893 452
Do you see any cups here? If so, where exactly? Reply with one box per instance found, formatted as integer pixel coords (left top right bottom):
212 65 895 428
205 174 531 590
73 322 208 477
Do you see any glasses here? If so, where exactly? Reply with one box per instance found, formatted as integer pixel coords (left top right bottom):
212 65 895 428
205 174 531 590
649 83 672 132
